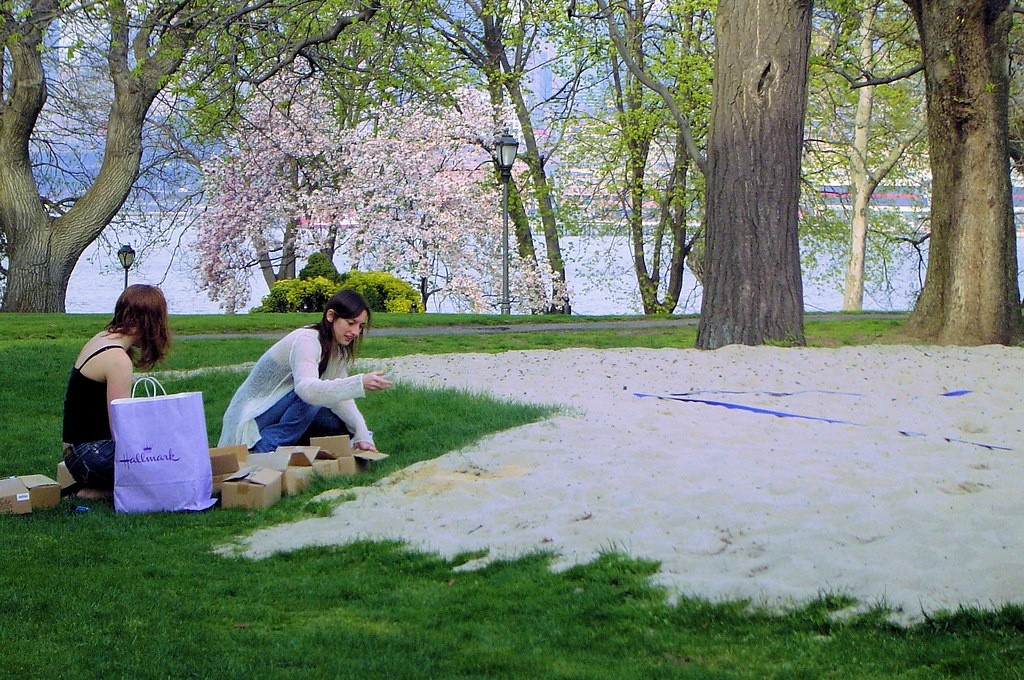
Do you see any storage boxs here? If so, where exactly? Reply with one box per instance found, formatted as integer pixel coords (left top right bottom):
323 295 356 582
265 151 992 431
208 435 390 511
0 461 111 517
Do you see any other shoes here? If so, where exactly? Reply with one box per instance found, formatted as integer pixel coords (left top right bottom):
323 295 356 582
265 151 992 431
76 487 113 501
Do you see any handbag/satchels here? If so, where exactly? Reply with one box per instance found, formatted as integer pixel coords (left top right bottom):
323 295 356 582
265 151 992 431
111 377 218 514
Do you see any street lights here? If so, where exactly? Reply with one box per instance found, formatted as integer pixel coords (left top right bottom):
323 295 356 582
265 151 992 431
116 245 137 289
492 130 521 318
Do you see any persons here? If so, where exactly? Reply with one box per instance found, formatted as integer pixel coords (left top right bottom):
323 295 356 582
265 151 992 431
218 289 393 454
61 284 173 500
